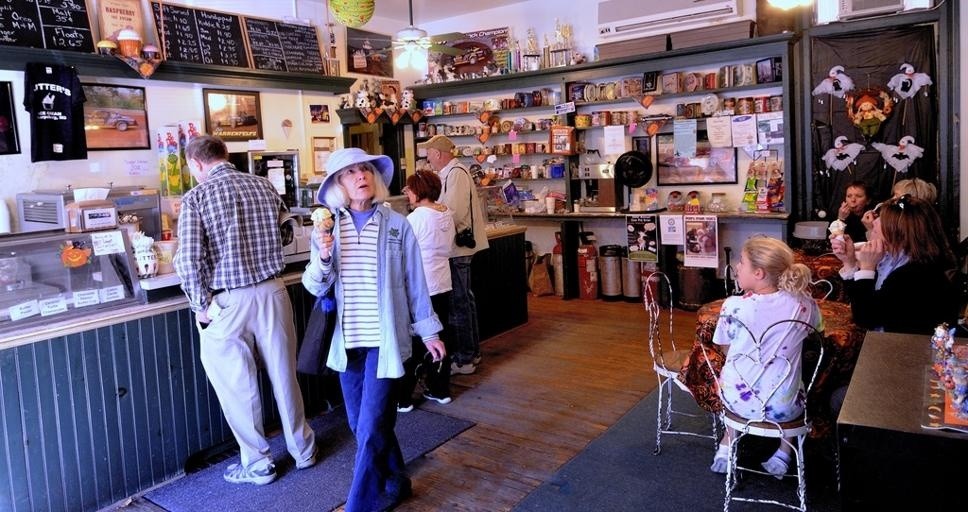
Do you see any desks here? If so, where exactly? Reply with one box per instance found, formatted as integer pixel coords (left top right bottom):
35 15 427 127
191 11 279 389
837 329 968 511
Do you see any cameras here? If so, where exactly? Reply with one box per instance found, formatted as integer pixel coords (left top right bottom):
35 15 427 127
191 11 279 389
454 226 476 249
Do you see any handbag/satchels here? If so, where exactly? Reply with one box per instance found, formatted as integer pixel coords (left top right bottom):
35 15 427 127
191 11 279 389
297 283 337 374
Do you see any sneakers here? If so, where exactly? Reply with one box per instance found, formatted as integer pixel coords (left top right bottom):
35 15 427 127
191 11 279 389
761 457 789 480
296 428 319 469
223 462 277 485
468 353 481 363
397 405 413 412
423 389 451 404
451 362 475 375
710 455 737 473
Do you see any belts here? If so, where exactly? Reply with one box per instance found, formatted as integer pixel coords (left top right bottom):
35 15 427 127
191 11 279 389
211 274 283 295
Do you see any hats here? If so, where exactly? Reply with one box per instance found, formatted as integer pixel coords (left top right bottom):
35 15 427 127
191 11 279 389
417 135 455 153
317 148 394 207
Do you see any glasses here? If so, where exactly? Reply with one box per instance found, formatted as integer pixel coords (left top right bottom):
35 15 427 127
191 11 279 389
415 170 424 181
912 175 919 200
897 193 912 210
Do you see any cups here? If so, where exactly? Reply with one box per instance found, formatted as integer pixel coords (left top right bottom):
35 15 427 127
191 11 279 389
545 198 555 215
453 142 550 157
630 194 639 210
418 113 566 138
500 161 565 178
162 229 172 239
424 88 560 116
156 241 176 274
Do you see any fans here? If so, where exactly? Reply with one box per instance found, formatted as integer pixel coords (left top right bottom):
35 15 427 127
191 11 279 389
345 0 468 60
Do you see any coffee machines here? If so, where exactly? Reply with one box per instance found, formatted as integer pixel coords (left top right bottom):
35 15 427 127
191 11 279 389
577 163 624 213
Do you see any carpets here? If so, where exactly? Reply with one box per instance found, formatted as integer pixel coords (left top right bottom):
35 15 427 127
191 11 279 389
508 334 967 511
141 399 479 512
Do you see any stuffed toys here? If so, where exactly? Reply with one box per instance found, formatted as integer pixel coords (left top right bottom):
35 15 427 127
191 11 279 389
336 76 416 110
426 60 504 85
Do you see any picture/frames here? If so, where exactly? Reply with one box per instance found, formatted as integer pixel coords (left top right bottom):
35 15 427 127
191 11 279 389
201 87 263 141
79 81 151 151
0 81 21 155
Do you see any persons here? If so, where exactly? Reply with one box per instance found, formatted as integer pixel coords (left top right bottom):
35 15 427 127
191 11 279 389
396 168 452 412
172 133 320 487
300 146 446 512
401 134 489 376
709 235 820 480
825 177 967 435
696 222 713 254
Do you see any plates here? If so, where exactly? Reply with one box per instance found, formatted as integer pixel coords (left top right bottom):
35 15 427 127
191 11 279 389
582 81 621 101
702 94 718 115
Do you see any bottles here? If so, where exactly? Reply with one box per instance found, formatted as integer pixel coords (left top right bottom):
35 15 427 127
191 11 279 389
573 111 640 130
677 95 783 118
574 200 579 212
708 192 731 212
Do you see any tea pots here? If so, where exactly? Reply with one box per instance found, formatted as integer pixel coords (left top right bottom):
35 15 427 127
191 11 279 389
583 149 601 165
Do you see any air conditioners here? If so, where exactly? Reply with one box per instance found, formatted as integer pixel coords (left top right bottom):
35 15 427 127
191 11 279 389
598 0 742 39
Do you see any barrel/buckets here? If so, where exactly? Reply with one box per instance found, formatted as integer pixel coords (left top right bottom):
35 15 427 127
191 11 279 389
675 251 717 312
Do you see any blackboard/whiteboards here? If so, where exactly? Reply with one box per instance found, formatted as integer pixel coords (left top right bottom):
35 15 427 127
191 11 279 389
242 14 328 76
148 0 252 69
0 0 99 55
